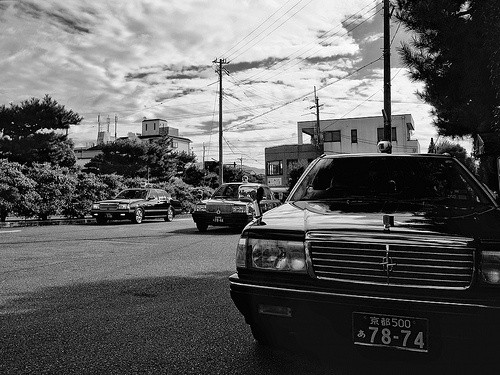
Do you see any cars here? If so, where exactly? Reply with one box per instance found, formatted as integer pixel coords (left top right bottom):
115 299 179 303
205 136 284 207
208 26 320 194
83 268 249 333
90 188 183 224
229 140 500 375
190 176 281 232
